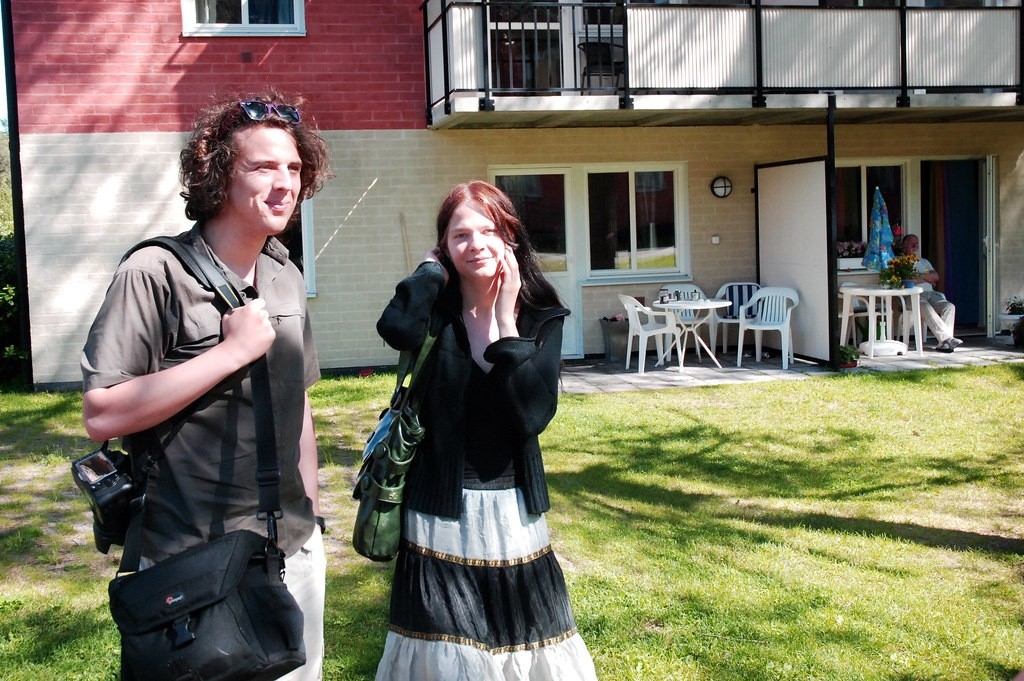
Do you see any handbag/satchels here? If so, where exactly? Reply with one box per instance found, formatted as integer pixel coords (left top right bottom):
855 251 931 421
108 528 307 681
353 409 423 562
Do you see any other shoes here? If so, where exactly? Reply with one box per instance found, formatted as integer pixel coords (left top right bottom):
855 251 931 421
936 343 954 352
949 338 963 348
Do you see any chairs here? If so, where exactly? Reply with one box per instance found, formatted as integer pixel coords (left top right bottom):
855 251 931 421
659 284 715 362
618 294 683 373
713 282 764 362
577 41 625 95
736 287 799 368
839 280 927 347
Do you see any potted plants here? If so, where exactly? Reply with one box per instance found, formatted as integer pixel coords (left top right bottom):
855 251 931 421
998 297 1024 345
838 344 860 368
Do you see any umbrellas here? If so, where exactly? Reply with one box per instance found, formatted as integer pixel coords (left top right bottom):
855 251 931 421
861 187 895 272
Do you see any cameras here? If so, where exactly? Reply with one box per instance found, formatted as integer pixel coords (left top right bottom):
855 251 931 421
69 449 141 555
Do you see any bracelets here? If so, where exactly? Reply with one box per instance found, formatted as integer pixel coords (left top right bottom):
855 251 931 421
316 516 325 534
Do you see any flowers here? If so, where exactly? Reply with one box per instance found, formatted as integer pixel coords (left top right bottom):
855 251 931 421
836 241 868 258
880 255 930 290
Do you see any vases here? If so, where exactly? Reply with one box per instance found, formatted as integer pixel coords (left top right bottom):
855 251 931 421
902 279 916 288
837 257 867 270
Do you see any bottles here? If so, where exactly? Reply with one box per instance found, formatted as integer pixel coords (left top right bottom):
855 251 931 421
659 289 668 304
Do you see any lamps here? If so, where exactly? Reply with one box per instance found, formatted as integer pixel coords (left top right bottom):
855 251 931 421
711 176 733 198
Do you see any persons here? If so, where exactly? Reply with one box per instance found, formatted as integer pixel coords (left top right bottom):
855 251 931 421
376 181 599 680
80 93 327 681
903 234 963 351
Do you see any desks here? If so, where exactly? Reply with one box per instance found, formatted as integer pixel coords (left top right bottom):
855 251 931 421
838 285 924 356
652 300 732 368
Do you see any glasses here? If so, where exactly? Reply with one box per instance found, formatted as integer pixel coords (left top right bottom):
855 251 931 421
215 100 301 138
